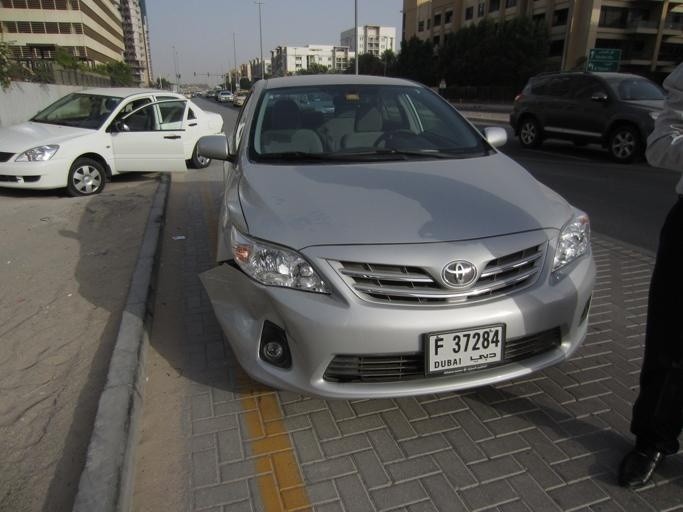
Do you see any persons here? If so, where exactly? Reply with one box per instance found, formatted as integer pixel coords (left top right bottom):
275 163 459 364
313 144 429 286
615 62 682 489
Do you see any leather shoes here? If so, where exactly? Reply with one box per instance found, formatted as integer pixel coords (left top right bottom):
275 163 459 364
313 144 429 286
619 440 665 489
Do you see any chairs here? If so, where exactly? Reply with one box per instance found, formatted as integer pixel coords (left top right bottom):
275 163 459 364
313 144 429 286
260 95 392 155
99 100 150 131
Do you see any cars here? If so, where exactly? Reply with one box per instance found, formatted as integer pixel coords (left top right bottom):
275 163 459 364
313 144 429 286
182 86 251 108
292 92 338 115
0 85 227 198
192 72 603 407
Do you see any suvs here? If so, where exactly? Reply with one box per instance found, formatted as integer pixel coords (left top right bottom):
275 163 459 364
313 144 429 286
507 70 666 162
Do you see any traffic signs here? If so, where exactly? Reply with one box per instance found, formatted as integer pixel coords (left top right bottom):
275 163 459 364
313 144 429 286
588 48 622 62
587 61 620 74
